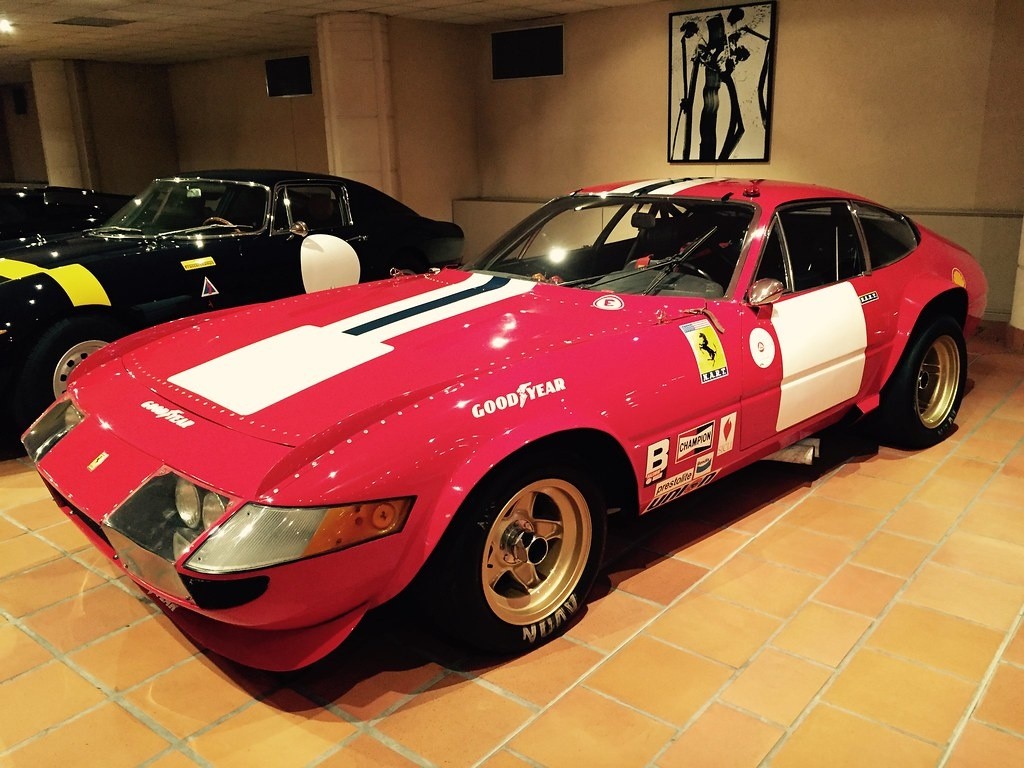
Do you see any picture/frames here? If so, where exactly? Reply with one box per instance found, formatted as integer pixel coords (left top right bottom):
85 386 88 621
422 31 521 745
666 0 777 164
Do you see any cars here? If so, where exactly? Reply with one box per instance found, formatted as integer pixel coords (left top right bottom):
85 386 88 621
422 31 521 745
0 169 465 448
20 178 989 671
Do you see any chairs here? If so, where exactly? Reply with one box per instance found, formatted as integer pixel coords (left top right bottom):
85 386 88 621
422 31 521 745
626 217 700 274
